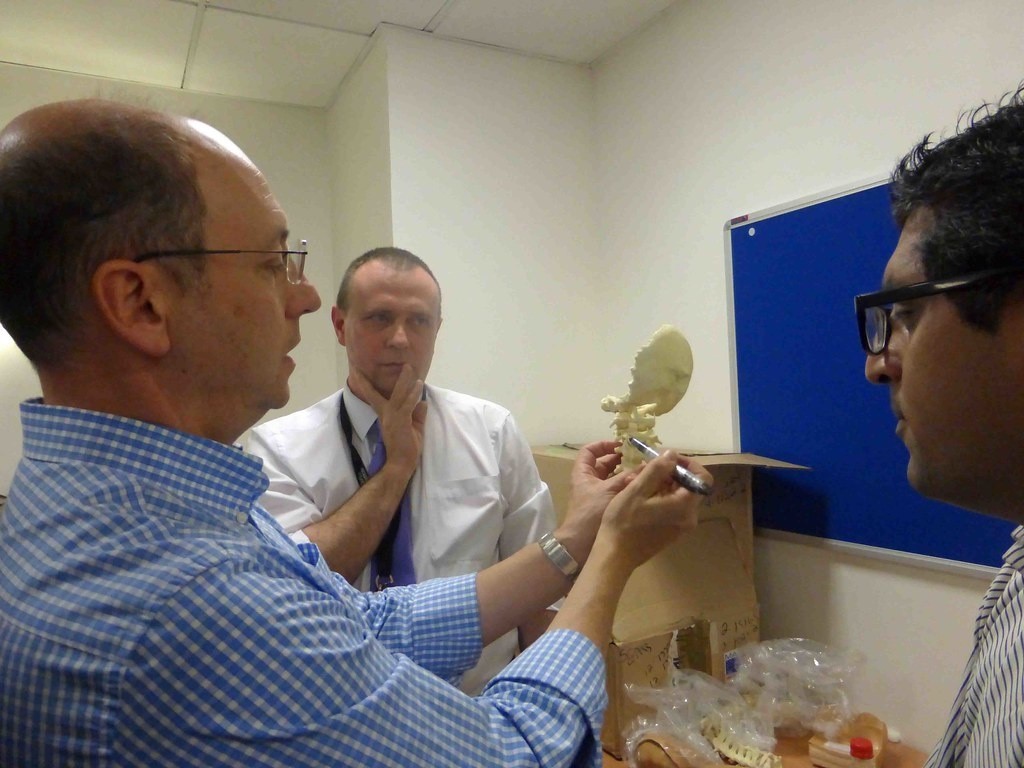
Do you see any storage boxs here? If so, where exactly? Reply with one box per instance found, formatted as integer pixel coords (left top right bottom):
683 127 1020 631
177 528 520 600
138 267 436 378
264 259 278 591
518 443 812 759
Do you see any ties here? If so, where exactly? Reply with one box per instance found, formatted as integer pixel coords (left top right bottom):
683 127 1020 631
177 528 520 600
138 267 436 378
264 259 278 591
368 414 416 591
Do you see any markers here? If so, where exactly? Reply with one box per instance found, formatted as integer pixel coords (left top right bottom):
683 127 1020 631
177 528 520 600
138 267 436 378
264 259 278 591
628 437 711 496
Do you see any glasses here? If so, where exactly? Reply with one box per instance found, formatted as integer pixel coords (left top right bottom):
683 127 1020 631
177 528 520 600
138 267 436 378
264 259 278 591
133 239 307 286
854 258 1023 355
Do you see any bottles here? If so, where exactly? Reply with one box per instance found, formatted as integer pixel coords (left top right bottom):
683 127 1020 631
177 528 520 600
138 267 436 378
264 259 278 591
848 738 878 768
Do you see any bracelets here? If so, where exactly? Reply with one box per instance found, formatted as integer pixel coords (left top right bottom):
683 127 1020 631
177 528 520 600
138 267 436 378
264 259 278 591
537 529 579 579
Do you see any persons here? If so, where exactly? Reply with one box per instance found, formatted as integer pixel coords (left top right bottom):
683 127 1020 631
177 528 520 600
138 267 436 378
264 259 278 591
855 85 1024 768
0 97 714 768
243 246 559 700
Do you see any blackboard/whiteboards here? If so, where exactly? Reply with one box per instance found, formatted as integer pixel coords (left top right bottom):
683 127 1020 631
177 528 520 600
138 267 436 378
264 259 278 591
724 178 1018 581
